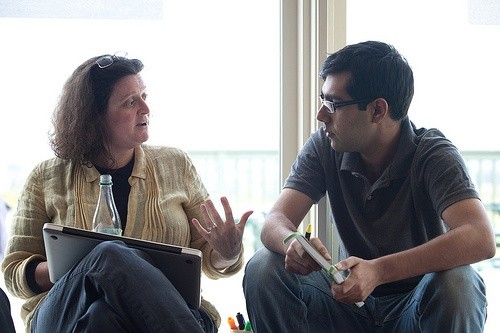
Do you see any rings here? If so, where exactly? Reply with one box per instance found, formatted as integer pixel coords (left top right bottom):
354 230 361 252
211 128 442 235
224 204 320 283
208 224 217 231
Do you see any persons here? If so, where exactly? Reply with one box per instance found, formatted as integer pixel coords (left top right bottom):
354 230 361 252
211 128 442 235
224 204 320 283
243 41 496 332
1 53 253 333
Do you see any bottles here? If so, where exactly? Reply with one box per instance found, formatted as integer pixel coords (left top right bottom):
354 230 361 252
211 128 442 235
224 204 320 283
92 174 122 235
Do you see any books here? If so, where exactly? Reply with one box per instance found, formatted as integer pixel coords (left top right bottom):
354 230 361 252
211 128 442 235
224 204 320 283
282 231 365 308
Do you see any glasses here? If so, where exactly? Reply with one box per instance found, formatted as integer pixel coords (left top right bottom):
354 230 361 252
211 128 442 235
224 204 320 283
319 94 369 113
96 50 129 68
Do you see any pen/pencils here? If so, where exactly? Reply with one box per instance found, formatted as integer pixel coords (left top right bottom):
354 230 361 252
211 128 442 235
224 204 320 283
227 317 237 329
305 224 311 240
236 312 245 330
246 322 252 331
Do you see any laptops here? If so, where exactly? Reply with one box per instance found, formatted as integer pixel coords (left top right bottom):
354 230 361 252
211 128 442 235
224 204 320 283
43 223 202 309
